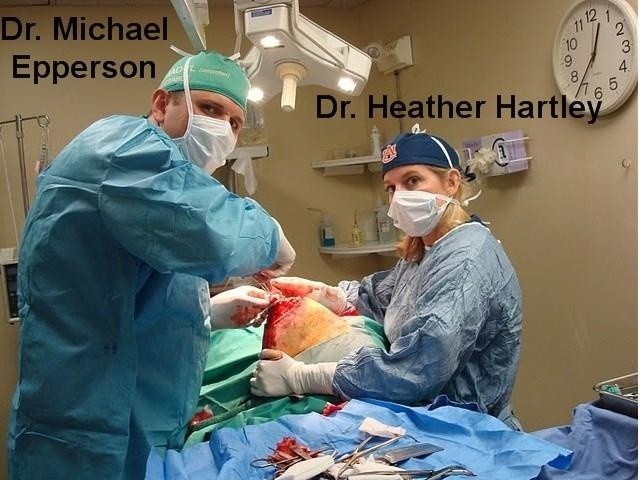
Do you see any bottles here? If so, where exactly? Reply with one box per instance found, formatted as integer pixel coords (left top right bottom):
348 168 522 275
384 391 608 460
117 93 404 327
319 216 336 248
373 195 396 245
352 221 364 245
368 125 383 156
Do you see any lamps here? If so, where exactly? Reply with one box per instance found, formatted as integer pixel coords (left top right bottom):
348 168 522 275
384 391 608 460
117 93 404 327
168 0 373 112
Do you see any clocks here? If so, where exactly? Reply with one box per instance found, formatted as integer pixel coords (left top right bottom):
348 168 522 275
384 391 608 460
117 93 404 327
551 0 638 116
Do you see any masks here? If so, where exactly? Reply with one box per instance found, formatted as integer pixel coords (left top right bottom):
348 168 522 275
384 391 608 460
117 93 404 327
169 89 237 177
386 189 452 238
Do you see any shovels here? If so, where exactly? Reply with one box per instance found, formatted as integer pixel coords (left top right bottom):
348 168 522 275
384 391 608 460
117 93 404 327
384 443 444 464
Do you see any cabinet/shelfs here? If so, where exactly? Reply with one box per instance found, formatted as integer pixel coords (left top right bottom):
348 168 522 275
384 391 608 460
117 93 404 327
312 154 407 255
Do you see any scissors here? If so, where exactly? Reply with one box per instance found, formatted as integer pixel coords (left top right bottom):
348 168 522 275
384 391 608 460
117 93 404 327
245 298 288 327
250 432 400 480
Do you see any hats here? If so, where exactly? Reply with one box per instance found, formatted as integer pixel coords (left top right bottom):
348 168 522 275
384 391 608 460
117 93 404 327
159 51 252 111
381 131 459 180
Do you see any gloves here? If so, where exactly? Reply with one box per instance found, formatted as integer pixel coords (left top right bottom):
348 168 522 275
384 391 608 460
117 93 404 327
271 276 347 316
250 348 338 399
208 286 271 330
254 219 296 284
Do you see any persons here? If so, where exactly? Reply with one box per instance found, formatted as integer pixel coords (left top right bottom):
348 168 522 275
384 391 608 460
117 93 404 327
6 53 294 480
247 134 520 418
